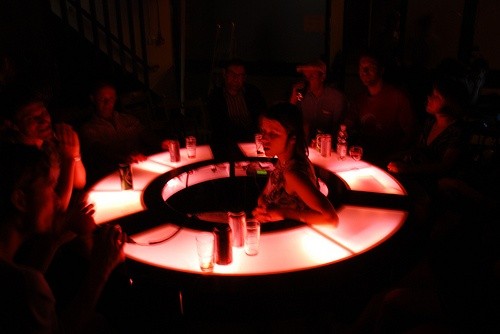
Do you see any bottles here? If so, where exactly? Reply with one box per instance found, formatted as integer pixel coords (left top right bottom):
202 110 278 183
228 205 247 249
336 124 348 160
213 223 234 265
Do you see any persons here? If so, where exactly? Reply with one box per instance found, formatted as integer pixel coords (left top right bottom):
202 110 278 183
289 62 343 141
0 95 128 334
343 53 414 164
386 85 456 173
250 103 338 223
78 82 144 180
208 59 262 131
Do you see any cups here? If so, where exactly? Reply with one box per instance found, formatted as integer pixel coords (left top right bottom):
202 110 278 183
185 136 197 158
255 134 265 156
243 220 260 255
196 233 216 273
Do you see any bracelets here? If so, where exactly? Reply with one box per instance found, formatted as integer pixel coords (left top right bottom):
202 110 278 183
75 156 80 162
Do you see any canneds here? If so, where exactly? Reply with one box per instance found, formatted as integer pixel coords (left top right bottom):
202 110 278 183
255 134 265 155
186 137 197 159
168 140 179 163
316 133 332 159
227 210 248 247
119 163 134 189
213 223 233 265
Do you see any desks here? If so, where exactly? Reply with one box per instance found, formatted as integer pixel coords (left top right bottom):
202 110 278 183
81 141 409 276
